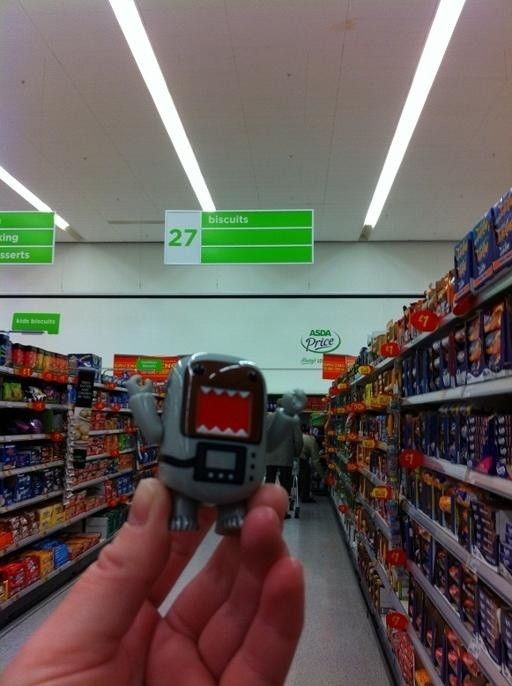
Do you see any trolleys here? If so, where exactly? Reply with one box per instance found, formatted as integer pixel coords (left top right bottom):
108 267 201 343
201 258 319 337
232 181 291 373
262 456 300 520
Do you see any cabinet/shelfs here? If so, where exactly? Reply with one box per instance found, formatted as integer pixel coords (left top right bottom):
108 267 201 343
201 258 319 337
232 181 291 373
314 246 511 686
1 364 169 629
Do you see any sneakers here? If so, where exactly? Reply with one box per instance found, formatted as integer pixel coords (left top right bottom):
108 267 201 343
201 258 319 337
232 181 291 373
301 497 316 503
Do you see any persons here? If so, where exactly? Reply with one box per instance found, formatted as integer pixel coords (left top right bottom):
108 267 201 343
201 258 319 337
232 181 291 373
265 404 325 519
0 472 305 686
